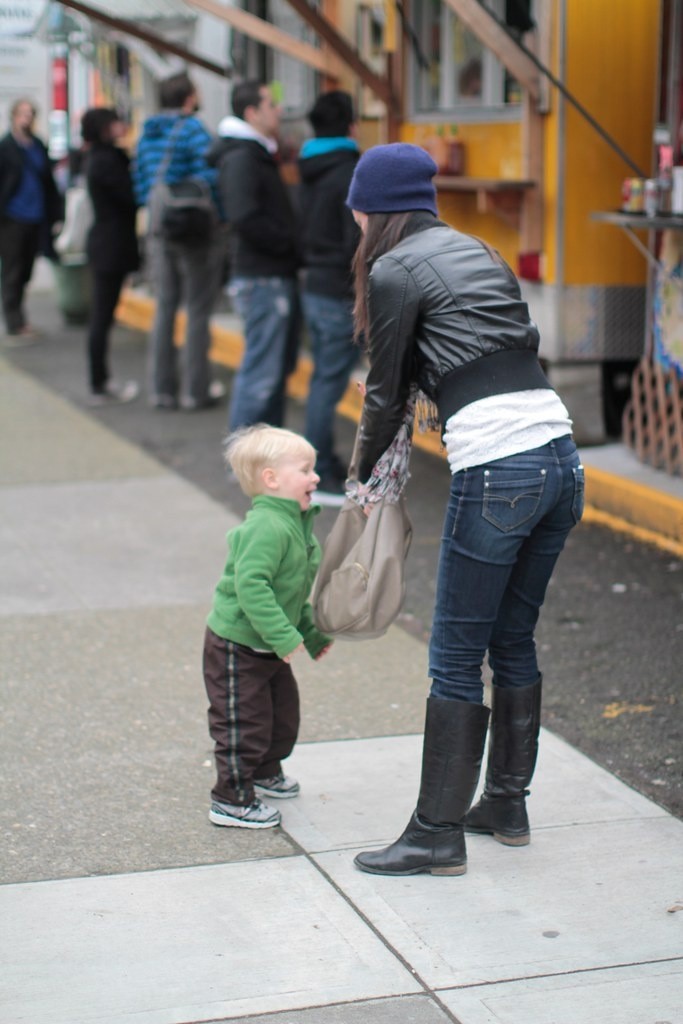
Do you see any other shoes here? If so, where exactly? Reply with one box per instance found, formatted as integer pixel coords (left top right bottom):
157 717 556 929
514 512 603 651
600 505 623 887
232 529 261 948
6 326 48 346
88 382 139 404
181 380 225 409
150 391 178 409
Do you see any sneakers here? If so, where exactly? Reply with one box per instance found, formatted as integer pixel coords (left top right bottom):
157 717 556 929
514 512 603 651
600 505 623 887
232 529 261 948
310 459 348 506
209 797 281 828
253 771 299 798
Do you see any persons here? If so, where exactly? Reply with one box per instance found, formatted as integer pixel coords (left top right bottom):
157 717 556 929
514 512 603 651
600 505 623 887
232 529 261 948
0 99 66 341
295 90 362 477
79 107 145 406
133 71 231 411
201 429 333 829
53 149 94 323
205 81 300 481
344 144 586 878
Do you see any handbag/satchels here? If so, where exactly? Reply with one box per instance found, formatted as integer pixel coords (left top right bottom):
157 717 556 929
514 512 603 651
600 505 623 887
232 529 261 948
53 187 96 254
152 181 222 239
312 495 413 641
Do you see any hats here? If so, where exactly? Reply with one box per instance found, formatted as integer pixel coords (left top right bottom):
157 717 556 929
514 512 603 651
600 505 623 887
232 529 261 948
345 144 438 216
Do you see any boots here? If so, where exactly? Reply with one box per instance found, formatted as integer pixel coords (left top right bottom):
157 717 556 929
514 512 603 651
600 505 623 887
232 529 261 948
463 671 542 846
354 699 492 876
